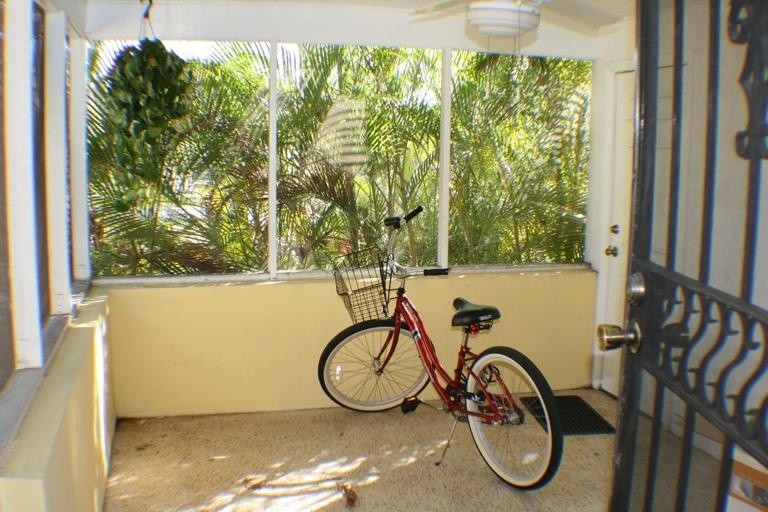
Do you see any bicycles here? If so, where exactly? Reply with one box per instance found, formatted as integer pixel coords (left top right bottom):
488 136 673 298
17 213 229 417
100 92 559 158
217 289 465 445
317 206 563 491
